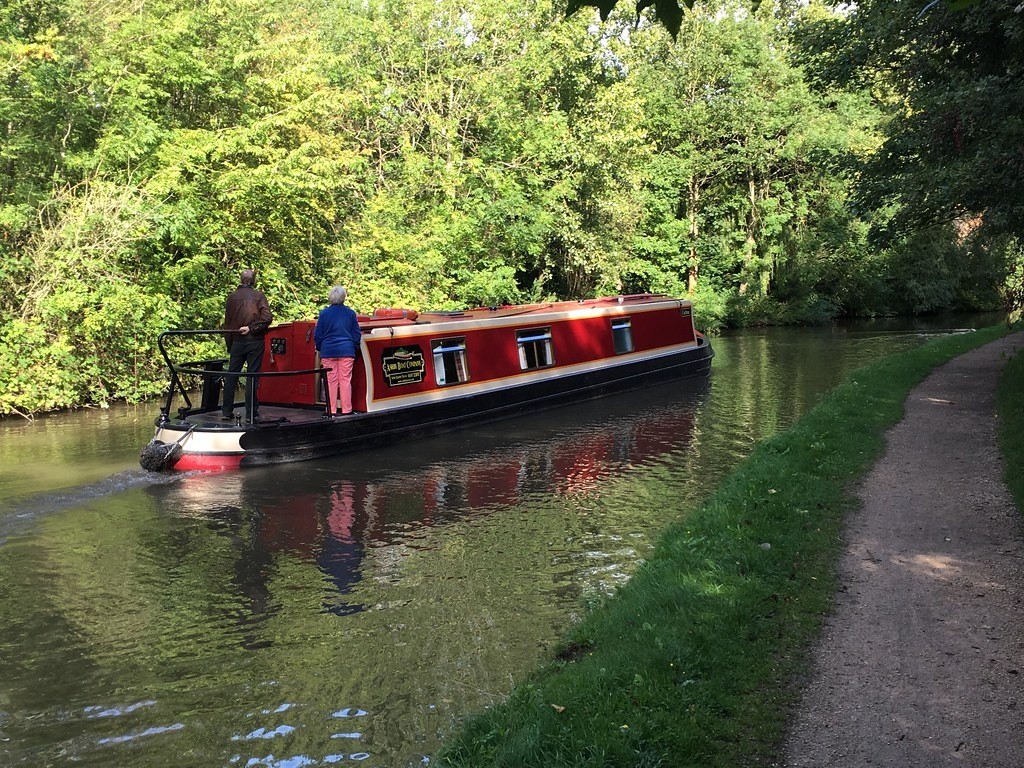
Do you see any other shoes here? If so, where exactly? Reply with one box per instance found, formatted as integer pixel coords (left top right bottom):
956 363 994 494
321 412 335 419
342 411 361 415
246 413 259 421
221 413 235 420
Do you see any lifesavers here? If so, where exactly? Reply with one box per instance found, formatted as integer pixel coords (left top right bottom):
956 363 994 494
373 307 419 320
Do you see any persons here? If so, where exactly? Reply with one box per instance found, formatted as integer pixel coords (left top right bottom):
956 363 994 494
314 287 361 420
220 268 273 421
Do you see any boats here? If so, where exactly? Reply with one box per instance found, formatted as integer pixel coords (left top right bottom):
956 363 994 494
140 293 716 473
